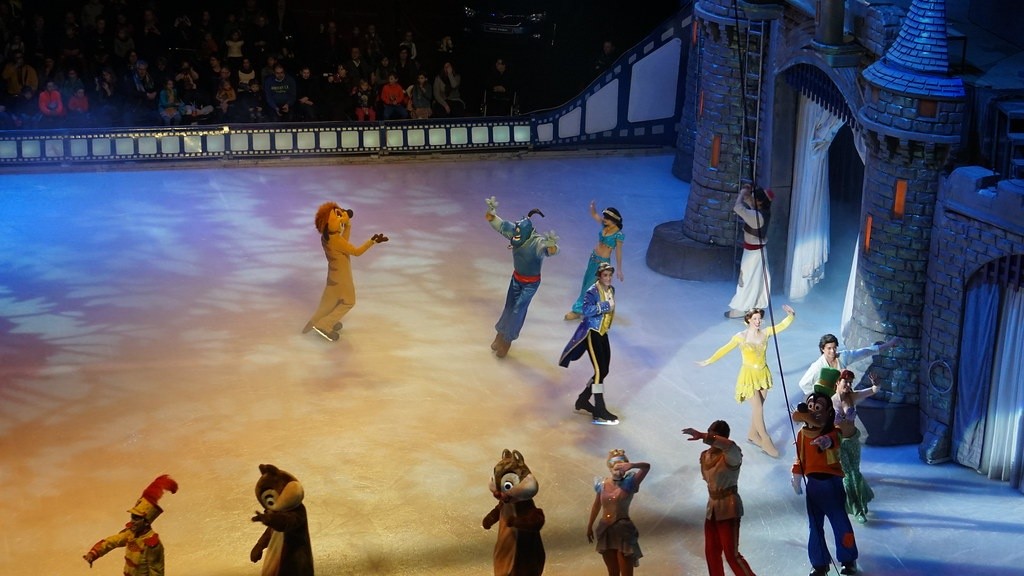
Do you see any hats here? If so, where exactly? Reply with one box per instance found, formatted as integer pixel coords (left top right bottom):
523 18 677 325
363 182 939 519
127 474 177 526
595 262 615 275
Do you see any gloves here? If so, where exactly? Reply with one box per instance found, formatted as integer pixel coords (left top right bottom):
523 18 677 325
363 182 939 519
810 436 831 451
485 196 499 216
608 299 616 311
792 474 803 495
541 230 560 248
372 234 389 244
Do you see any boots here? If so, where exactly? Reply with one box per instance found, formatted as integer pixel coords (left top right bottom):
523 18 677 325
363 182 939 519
574 379 595 416
590 383 619 426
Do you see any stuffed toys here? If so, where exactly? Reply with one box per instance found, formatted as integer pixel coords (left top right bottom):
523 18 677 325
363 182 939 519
483 449 546 576
251 465 314 576
302 201 389 341
792 367 858 576
486 196 560 357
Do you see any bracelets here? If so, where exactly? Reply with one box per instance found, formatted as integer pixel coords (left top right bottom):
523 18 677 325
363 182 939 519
703 433 716 444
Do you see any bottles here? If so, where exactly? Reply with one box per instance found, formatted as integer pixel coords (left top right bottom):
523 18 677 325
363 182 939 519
790 475 803 495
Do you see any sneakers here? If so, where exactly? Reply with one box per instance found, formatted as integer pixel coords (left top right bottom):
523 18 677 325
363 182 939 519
838 559 857 576
496 341 511 358
748 431 763 448
312 325 339 342
855 504 868 526
724 308 750 320
333 320 343 332
807 567 828 576
491 334 504 352
564 311 580 322
762 436 780 459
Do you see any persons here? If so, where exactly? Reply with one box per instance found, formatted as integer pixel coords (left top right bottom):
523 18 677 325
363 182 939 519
799 334 902 441
564 200 624 320
587 449 651 576
559 262 619 425
832 370 881 523
695 305 795 457
596 41 619 74
0 1 515 129
83 474 179 576
682 420 756 576
724 182 774 317
